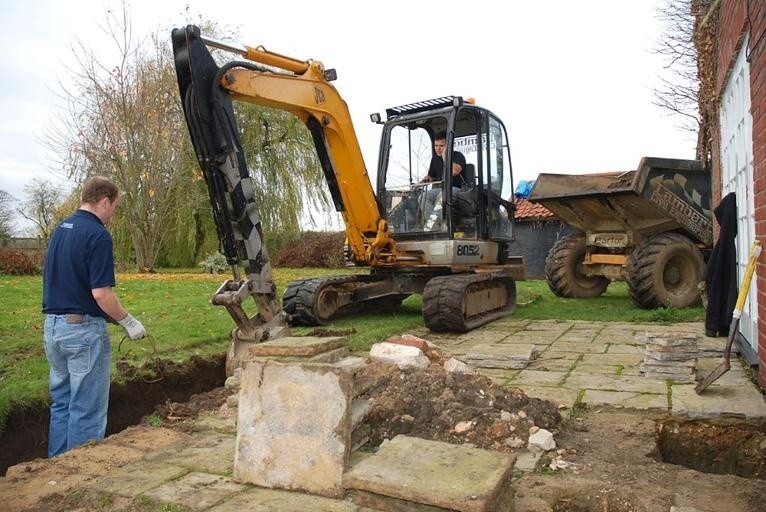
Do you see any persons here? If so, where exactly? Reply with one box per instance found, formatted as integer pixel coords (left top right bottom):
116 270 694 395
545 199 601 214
41 175 146 459
417 131 466 230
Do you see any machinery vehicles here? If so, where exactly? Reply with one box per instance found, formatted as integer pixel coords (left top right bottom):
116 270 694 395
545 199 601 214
166 21 530 381
524 155 714 312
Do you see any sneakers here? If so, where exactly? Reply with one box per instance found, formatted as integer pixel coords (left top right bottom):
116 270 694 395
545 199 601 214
437 223 448 233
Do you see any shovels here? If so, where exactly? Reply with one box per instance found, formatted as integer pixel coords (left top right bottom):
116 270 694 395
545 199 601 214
694 240 762 395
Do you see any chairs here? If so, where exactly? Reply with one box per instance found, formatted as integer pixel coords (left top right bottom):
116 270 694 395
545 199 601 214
433 163 476 213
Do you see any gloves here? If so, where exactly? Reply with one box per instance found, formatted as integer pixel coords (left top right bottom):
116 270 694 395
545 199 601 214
117 312 148 341
